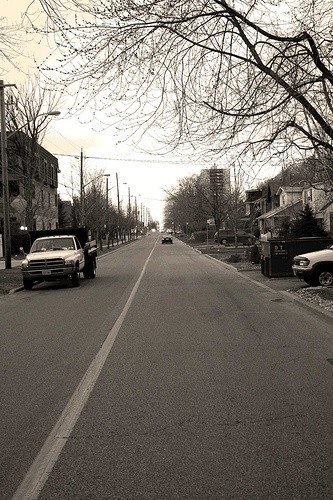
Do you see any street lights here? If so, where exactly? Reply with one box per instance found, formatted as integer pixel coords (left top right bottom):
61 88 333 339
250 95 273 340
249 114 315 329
116 172 133 238
0 80 61 269
106 177 127 244
80 148 110 228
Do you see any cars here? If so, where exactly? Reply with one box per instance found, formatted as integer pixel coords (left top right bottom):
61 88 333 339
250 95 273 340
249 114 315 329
291 245 333 286
214 229 256 245
162 234 173 244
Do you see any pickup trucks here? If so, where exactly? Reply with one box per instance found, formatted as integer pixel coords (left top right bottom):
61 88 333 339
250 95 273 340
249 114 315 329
19 234 99 290
